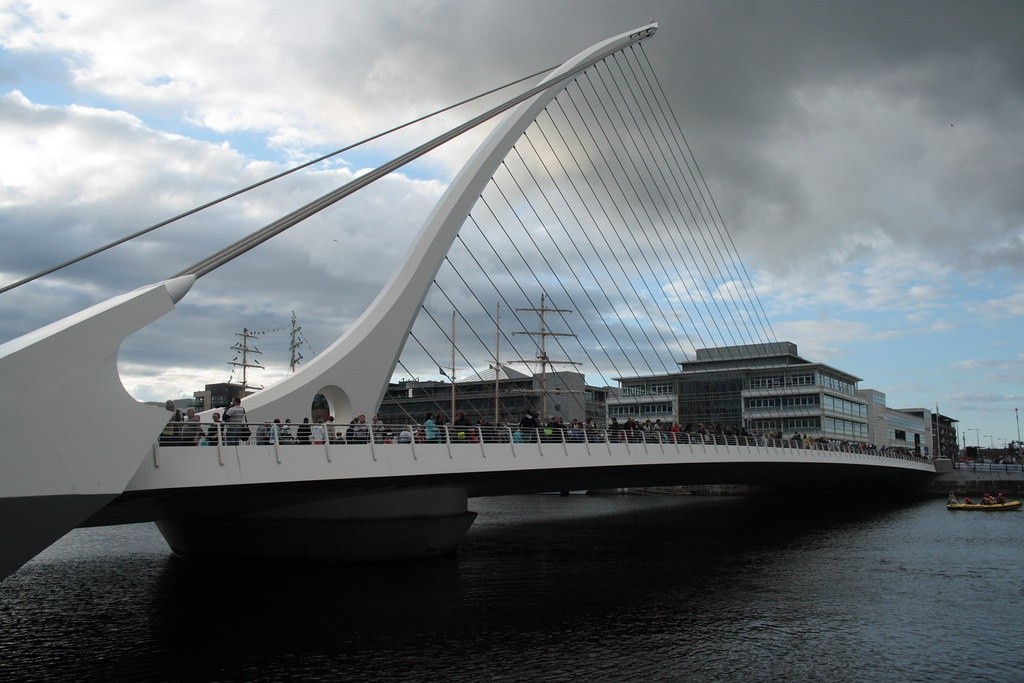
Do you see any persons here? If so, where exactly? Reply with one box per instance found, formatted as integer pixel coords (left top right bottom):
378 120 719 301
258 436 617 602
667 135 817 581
160 400 749 446
980 493 1006 505
947 491 957 504
749 429 934 466
965 498 972 504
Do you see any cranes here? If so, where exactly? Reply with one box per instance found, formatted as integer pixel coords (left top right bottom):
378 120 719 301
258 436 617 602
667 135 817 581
967 427 1007 449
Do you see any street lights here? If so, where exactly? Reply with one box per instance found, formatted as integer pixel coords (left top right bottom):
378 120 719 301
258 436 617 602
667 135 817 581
1015 407 1021 449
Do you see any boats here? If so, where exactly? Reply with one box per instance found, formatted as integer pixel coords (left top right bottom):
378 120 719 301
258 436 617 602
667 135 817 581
946 489 1023 512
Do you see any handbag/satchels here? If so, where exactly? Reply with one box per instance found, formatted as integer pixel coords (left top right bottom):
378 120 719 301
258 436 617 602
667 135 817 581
544 428 552 435
458 431 465 440
512 430 522 443
238 424 251 441
199 436 208 446
